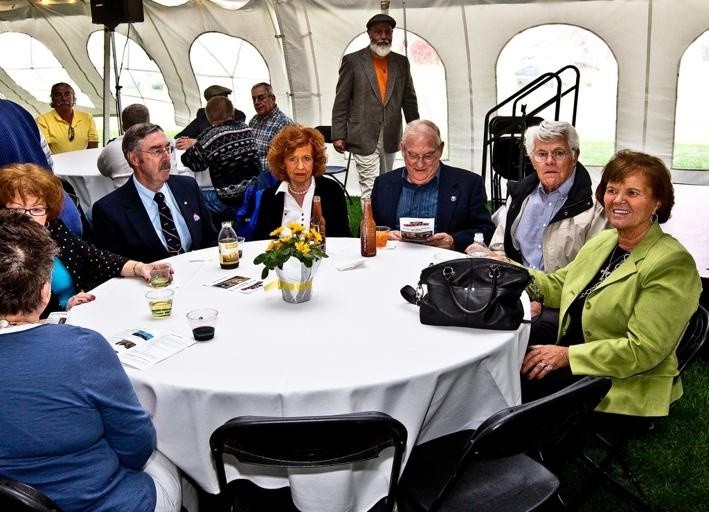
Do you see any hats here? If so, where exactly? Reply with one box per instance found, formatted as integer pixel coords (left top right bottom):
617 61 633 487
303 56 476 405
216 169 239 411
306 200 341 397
366 14 396 29
204 85 232 101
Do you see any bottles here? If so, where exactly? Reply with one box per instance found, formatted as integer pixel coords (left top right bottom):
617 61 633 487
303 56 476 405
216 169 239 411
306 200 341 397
359 196 378 257
309 195 326 254
217 220 240 269
466 232 490 260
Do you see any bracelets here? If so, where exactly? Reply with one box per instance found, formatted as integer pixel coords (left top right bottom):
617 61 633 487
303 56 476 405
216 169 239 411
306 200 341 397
133 261 145 277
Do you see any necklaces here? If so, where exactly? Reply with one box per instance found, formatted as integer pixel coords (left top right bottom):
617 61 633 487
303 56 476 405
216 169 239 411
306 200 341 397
598 242 634 281
1 315 37 329
287 183 310 196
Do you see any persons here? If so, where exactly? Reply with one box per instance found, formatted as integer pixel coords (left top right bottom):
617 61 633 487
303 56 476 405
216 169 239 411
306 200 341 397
331 13 420 216
36 82 99 155
174 84 246 139
1 213 201 512
97 104 177 191
247 82 295 158
371 119 496 250
466 119 616 327
92 123 220 263
1 162 174 319
0 98 54 173
181 96 262 215
464 148 702 417
251 124 354 240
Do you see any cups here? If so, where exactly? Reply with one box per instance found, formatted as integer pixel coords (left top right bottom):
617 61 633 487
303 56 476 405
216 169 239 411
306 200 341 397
375 225 391 248
236 237 245 258
148 262 171 288
184 308 219 342
143 289 176 320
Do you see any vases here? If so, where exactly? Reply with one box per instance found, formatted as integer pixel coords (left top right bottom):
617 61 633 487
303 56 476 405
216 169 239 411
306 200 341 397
275 258 313 303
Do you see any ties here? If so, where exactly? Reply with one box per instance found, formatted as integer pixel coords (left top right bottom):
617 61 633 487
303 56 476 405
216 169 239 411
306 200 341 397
153 192 185 256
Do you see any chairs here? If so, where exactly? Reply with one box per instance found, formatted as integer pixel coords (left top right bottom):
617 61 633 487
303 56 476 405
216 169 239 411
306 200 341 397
561 304 708 505
314 125 354 207
209 409 410 511
489 116 544 212
0 473 62 512
401 375 614 511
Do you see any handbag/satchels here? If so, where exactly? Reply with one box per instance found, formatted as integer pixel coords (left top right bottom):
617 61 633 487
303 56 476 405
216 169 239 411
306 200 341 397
400 258 544 332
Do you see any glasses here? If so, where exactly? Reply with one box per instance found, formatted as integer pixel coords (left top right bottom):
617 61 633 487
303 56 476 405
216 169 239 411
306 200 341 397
6 208 48 216
68 124 74 142
534 148 568 163
404 145 440 162
137 145 173 156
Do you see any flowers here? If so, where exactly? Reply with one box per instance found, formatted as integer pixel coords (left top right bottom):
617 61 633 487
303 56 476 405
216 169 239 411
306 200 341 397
253 223 329 280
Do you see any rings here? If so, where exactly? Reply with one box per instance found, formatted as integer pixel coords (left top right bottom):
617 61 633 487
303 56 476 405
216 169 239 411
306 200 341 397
538 361 545 368
